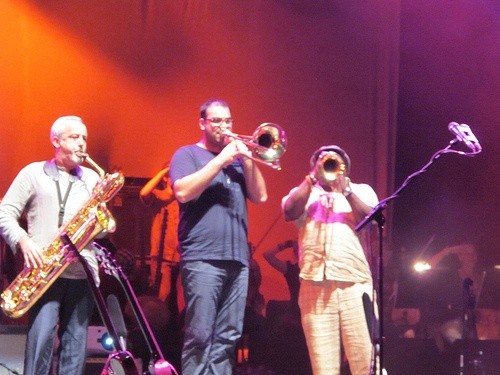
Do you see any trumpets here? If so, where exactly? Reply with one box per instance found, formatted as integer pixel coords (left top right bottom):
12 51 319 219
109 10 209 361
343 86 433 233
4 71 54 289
220 122 289 171
318 153 346 182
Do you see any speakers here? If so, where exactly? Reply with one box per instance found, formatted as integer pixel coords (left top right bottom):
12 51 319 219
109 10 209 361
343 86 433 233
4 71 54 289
0 325 28 375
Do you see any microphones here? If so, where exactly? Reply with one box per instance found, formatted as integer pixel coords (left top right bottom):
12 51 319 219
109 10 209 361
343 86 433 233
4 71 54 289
448 122 475 149
461 124 482 151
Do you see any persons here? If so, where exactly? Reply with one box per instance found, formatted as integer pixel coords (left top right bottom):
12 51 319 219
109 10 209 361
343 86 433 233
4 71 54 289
0 116 107 375
458 278 478 340
140 162 301 375
169 98 268 375
282 144 389 375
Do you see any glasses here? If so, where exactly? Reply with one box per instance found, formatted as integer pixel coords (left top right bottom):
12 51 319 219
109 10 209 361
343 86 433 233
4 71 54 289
205 118 233 123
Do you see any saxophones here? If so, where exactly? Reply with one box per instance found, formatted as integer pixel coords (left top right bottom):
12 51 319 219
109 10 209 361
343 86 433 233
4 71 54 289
0 153 125 319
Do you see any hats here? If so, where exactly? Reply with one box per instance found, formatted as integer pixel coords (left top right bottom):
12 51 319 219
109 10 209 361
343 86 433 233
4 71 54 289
313 145 351 173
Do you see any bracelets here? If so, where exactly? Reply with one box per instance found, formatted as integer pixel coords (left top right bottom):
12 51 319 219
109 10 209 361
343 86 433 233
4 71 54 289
305 173 318 186
341 186 357 203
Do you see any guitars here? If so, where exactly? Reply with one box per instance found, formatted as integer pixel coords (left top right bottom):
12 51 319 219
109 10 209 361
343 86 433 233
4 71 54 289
91 241 182 375
58 232 143 375
